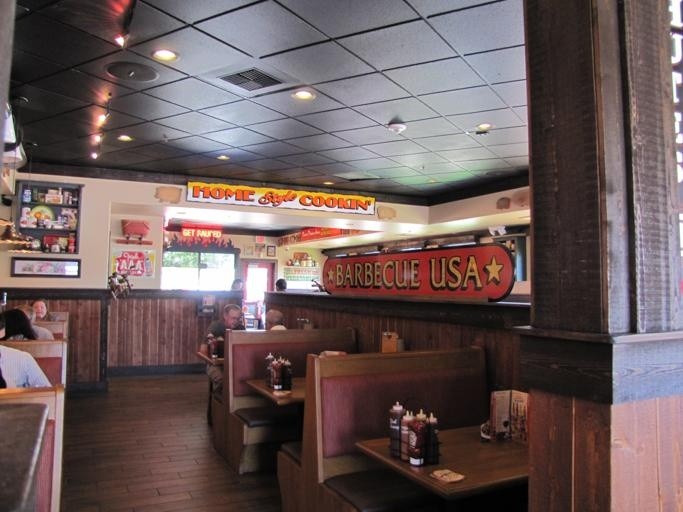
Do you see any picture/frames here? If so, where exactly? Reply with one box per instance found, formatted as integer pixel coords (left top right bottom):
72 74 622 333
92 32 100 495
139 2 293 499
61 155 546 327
11 257 80 277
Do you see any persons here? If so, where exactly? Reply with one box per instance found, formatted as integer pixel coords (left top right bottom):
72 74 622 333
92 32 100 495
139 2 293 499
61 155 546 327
0 305 54 341
0 308 38 342
230 277 244 290
32 300 56 322
198 303 245 400
274 278 288 291
0 345 53 390
262 308 288 330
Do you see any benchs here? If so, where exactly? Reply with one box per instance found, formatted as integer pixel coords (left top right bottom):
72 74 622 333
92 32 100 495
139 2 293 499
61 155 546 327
0 312 69 512
276 347 487 511
212 328 361 476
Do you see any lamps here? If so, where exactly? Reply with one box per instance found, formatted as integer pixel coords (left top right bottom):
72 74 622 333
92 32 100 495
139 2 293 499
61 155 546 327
91 112 111 159
115 32 131 48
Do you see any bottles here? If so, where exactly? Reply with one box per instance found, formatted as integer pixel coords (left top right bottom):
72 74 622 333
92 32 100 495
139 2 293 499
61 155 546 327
21 189 32 203
265 353 291 392
388 402 441 467
207 336 225 359
286 256 313 266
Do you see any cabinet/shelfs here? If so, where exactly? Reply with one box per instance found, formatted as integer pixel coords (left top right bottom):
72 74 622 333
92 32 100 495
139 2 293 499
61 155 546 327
10 180 85 253
